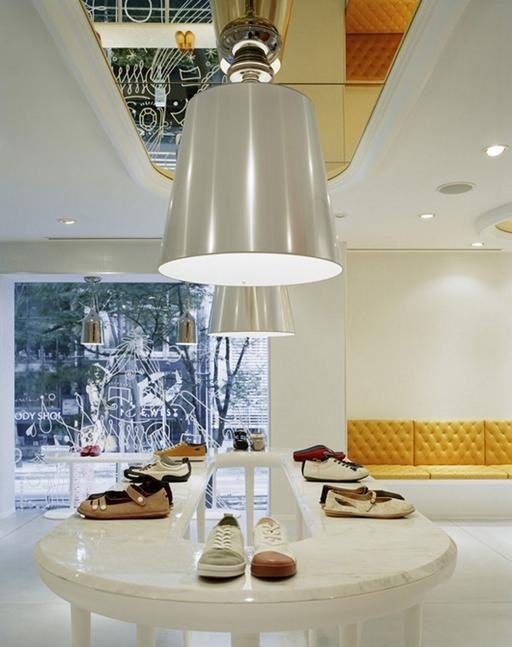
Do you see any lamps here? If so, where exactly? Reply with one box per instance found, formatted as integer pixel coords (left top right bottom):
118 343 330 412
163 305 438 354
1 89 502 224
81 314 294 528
206 284 296 339
80 277 103 346
158 0 344 287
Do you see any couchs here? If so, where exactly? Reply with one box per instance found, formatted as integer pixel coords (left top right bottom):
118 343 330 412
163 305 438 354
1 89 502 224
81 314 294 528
347 418 512 521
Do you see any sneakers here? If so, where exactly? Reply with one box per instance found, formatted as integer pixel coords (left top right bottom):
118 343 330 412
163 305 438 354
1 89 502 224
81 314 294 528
293 444 369 482
233 432 266 451
76 441 207 520
197 513 247 578
249 518 298 578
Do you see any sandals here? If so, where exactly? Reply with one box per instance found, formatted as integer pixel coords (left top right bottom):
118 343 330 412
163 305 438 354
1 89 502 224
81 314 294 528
319 484 415 518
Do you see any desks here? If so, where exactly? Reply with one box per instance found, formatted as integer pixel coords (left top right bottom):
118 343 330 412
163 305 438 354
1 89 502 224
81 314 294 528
81 1 217 51
45 452 152 520
